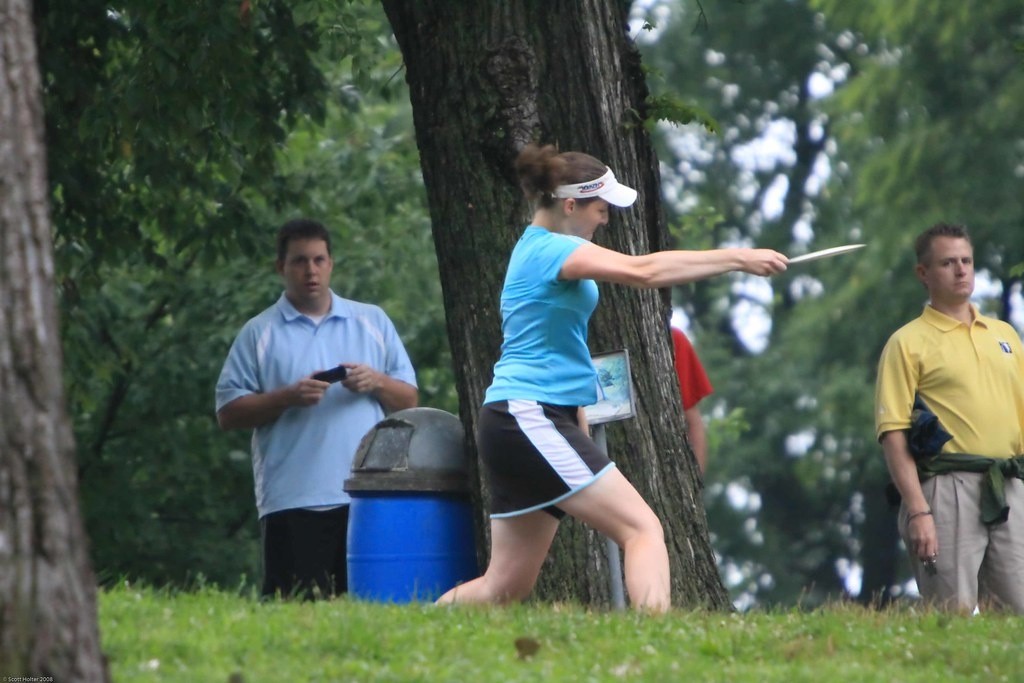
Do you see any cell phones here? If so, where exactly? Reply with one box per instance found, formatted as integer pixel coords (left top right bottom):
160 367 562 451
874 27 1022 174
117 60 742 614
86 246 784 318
313 366 348 383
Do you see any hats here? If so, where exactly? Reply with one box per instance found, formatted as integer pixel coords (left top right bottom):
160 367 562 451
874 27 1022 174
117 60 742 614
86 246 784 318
542 165 637 208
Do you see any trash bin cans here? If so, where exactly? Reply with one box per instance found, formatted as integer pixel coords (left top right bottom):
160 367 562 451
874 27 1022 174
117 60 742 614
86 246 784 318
337 404 483 605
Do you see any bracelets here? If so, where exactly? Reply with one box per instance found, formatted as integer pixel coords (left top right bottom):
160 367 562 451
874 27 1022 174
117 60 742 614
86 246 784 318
907 510 933 524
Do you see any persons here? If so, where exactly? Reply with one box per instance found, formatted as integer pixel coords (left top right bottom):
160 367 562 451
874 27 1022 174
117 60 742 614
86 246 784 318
215 219 421 598
437 145 790 617
874 221 1024 615
669 326 713 474
